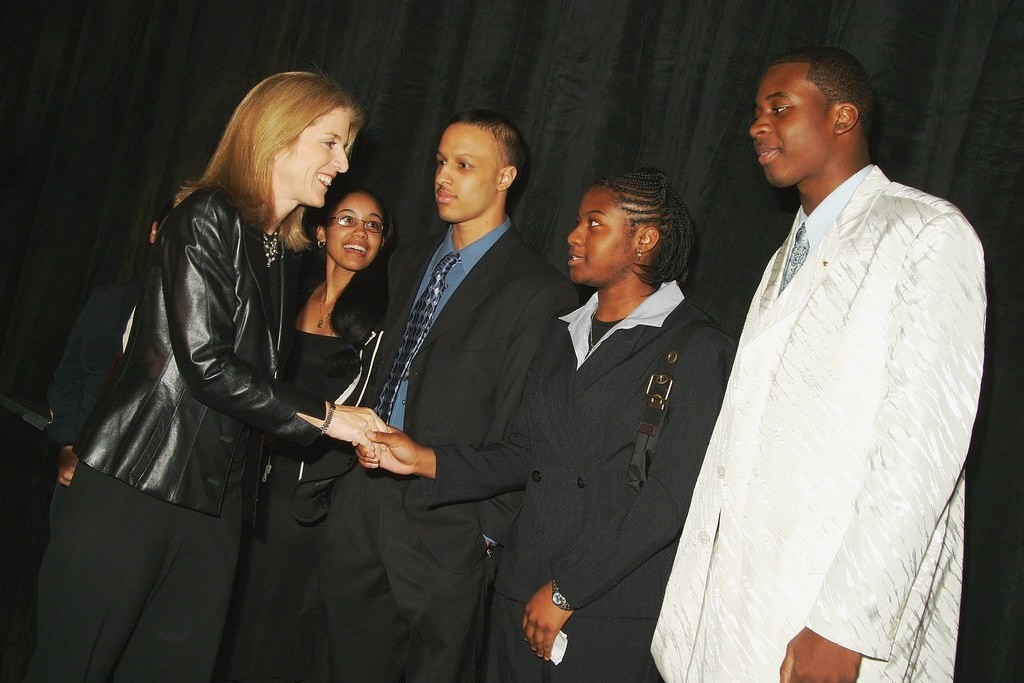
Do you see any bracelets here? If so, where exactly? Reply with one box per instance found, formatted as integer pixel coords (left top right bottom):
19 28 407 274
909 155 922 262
320 400 335 435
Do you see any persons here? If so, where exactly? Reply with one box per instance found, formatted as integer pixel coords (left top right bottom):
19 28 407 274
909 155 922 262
23 70 391 683
40 110 733 683
650 44 988 683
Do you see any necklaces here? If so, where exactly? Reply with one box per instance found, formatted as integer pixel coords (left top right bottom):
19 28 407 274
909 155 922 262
317 282 342 328
260 232 279 266
589 307 631 350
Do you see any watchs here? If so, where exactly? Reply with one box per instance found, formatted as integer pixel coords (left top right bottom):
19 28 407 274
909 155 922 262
552 578 573 611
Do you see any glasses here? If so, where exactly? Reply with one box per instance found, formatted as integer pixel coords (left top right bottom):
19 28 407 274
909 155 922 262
328 215 385 234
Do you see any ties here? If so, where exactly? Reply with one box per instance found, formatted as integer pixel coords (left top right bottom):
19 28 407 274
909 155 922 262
376 251 463 427
779 222 809 295
